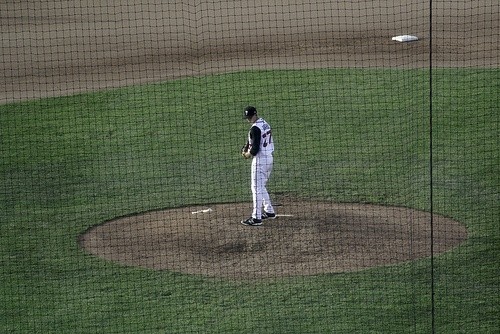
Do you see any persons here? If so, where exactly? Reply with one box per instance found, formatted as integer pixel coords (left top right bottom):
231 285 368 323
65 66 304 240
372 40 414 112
241 107 276 225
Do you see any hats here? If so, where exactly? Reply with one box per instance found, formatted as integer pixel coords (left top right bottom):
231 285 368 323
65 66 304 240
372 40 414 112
242 106 257 120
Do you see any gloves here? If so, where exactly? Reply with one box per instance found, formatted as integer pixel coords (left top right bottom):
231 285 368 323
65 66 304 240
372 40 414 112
244 152 252 159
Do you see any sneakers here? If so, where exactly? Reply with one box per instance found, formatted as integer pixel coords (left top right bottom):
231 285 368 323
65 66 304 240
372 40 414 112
241 217 263 226
261 211 276 221
